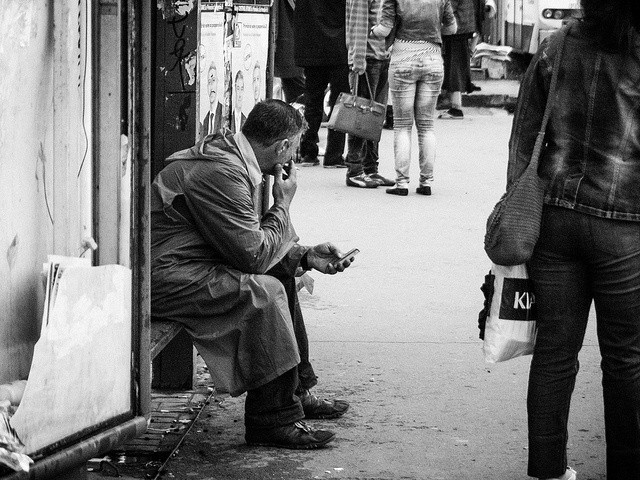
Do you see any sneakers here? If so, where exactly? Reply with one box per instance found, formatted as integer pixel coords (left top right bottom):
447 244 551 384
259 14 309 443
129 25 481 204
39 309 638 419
545 466 576 480
368 173 395 187
345 173 378 188
300 388 349 418
245 420 335 448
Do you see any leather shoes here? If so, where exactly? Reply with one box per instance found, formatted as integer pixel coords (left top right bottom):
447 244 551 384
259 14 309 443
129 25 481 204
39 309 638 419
301 156 319 167
323 158 347 168
385 186 408 195
416 186 432 196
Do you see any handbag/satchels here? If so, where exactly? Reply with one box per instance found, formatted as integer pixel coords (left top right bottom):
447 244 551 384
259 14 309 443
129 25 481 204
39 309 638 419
483 20 573 265
326 69 385 143
478 255 538 365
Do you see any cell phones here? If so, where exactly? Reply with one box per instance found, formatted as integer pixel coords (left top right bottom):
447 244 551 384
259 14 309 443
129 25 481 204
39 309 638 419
331 247 360 269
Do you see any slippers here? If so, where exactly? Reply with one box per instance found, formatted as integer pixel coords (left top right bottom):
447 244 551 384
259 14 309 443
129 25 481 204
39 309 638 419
438 111 463 120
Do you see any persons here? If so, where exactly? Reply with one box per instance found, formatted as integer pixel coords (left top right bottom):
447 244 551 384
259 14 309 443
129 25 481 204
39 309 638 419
370 0 457 197
507 1 640 480
251 66 263 104
345 0 396 189
275 1 307 106
152 99 352 449
231 70 247 135
203 63 222 137
293 0 350 168
442 1 480 94
437 34 465 119
